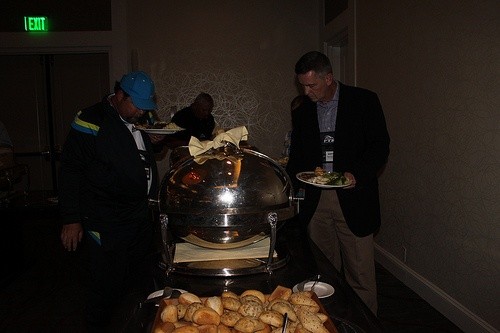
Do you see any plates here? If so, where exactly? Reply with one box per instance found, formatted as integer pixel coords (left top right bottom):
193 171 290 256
138 128 185 134
151 291 338 333
147 289 188 307
293 281 334 298
296 172 352 188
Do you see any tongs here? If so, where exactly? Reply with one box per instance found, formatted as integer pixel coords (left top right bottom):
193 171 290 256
138 287 181 309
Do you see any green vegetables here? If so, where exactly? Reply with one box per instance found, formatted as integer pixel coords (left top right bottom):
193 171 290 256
321 172 346 185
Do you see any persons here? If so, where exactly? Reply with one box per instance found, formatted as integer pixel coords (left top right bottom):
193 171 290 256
53 70 166 333
285 51 392 319
167 92 215 167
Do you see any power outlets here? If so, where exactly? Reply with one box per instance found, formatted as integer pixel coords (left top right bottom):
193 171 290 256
401 244 407 263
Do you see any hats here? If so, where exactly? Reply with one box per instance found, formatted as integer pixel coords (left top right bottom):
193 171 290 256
120 70 156 110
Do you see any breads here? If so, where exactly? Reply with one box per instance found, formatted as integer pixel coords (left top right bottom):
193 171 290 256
155 286 329 333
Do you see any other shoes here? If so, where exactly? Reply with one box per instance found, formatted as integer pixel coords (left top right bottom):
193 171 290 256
87 324 107 333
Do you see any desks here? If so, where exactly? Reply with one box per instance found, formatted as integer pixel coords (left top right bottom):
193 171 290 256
146 218 389 333
